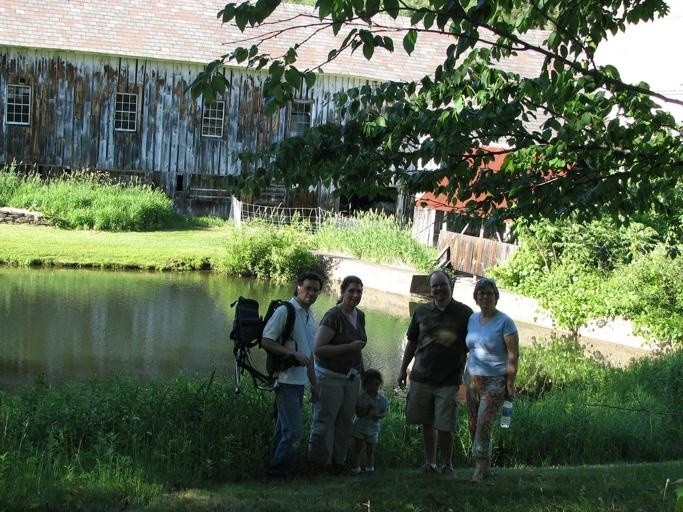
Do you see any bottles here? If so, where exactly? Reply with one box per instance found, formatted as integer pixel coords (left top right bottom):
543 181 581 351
501 399 512 430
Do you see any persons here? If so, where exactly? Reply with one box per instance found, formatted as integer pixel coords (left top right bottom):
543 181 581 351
348 368 388 476
306 275 368 478
397 270 474 475
260 273 322 474
463 278 520 484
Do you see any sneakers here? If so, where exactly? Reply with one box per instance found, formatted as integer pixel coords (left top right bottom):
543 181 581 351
269 466 376 476
419 463 454 475
